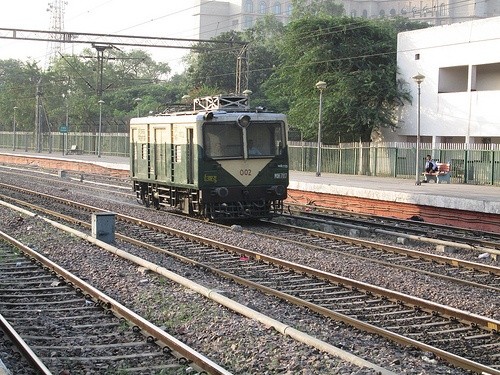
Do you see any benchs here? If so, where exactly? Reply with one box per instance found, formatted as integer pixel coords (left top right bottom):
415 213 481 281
422 161 452 184
68 145 82 154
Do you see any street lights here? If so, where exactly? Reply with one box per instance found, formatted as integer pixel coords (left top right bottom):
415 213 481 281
97 99 105 158
314 80 326 177
134 98 142 118
412 73 425 183
13 106 18 151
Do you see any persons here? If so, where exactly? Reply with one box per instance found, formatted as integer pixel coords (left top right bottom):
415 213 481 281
425 154 438 175
247 138 262 156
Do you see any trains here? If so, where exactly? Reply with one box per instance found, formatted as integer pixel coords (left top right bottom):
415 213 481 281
128 94 290 223
